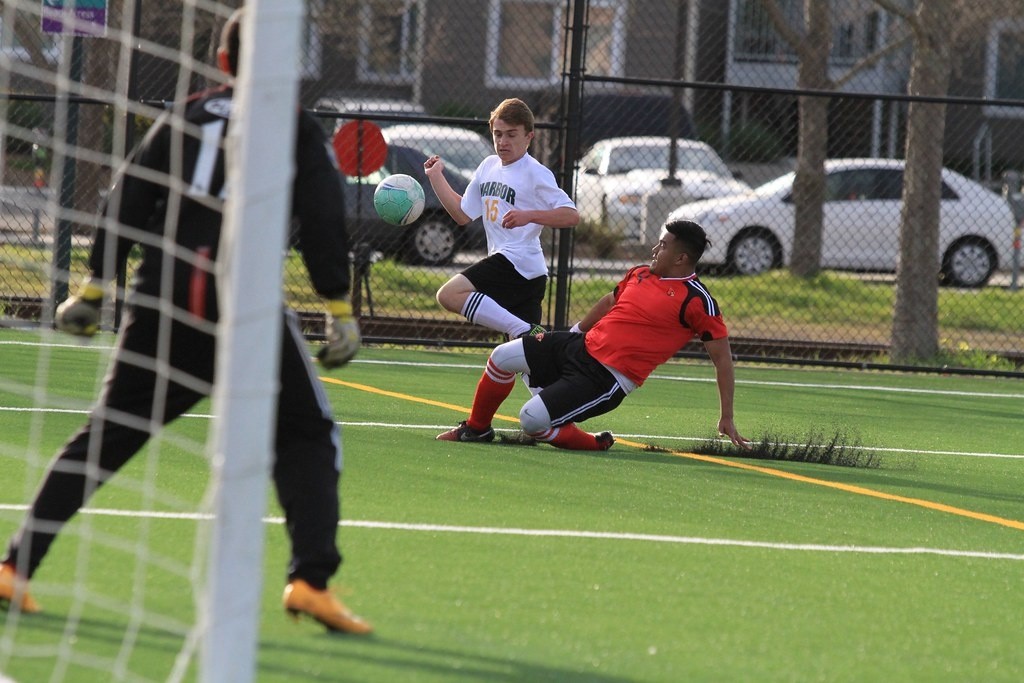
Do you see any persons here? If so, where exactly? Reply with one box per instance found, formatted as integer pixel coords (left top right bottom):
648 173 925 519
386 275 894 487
424 98 579 396
0 6 370 635
436 220 750 450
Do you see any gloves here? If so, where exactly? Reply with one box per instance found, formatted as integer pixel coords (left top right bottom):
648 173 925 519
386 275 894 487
55 276 103 338
315 301 360 369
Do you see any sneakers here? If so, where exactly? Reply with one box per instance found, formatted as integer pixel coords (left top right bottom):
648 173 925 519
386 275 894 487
595 432 614 450
284 580 371 636
0 563 36 612
435 420 495 442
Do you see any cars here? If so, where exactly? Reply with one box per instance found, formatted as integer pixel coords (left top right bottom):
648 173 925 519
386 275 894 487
311 95 499 268
656 155 1024 289
568 134 757 255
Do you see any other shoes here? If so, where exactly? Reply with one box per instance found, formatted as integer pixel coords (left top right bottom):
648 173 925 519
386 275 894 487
514 324 546 339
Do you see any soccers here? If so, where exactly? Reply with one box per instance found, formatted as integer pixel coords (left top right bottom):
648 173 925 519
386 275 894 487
374 174 425 226
